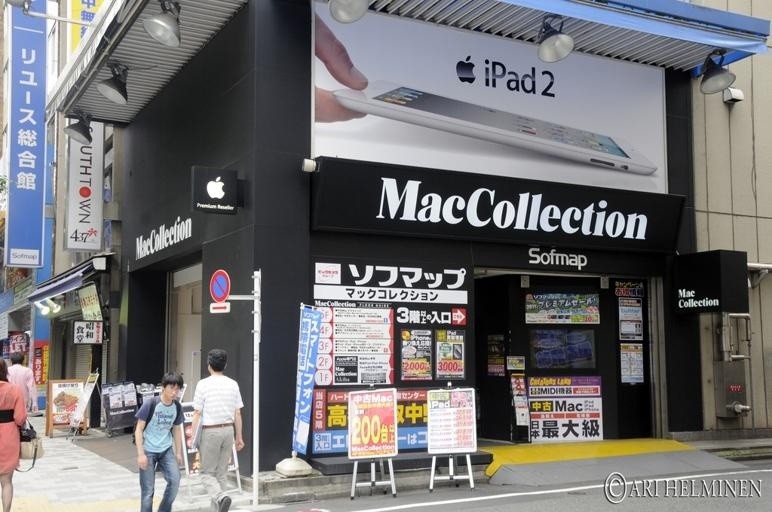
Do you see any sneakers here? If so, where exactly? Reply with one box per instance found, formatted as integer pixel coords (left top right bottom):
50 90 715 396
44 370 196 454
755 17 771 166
218 496 232 512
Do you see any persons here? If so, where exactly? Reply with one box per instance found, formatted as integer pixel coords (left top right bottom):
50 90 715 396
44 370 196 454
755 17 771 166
134 371 186 512
0 358 27 512
6 351 38 430
191 349 246 512
314 12 369 123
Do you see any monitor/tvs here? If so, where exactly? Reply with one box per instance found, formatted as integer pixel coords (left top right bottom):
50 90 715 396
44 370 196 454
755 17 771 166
520 286 601 329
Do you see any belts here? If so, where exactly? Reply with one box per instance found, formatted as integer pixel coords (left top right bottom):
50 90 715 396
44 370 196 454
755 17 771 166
201 422 233 430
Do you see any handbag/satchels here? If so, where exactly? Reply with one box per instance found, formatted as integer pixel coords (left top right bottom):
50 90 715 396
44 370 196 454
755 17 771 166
19 418 45 460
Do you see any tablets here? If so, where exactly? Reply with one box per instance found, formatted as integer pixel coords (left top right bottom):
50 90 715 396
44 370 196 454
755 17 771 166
333 78 658 177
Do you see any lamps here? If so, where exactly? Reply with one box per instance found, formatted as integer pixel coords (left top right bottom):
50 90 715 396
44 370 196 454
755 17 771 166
142 0 188 48
698 44 739 94
532 9 574 66
95 59 129 105
62 108 93 144
